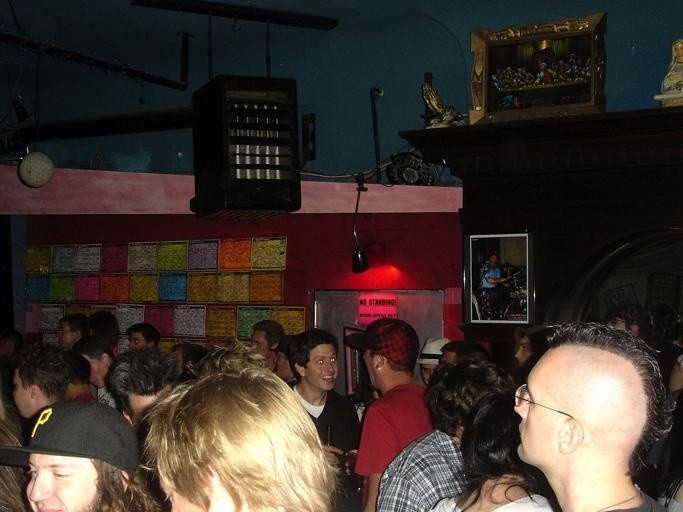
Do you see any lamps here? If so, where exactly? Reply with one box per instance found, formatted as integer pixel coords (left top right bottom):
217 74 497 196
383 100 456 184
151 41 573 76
351 171 369 273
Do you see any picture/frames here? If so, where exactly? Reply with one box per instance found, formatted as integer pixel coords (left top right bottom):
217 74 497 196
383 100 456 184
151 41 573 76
463 225 537 331
469 7 608 126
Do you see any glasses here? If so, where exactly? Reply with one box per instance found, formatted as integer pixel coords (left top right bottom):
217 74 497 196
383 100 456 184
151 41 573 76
510 384 577 424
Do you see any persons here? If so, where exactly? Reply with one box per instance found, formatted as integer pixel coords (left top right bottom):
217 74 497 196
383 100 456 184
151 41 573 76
106 348 179 424
440 341 489 367
374 357 516 512
606 302 654 336
414 336 451 385
0 402 161 512
251 320 284 370
126 323 161 352
11 343 68 419
268 334 297 382
285 328 365 511
60 350 96 403
71 336 118 410
144 368 340 512
56 313 89 350
516 324 549 374
479 251 507 321
86 311 120 351
514 321 677 512
0 331 24 365
430 387 554 512
343 318 431 512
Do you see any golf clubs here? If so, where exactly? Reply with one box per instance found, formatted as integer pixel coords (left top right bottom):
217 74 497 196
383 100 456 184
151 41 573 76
370 85 385 185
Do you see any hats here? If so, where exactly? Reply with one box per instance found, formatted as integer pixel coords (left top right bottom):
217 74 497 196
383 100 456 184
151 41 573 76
2 400 140 475
343 318 418 368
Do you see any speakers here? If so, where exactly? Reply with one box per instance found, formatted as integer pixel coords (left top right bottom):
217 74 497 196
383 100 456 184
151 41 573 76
193 74 301 219
